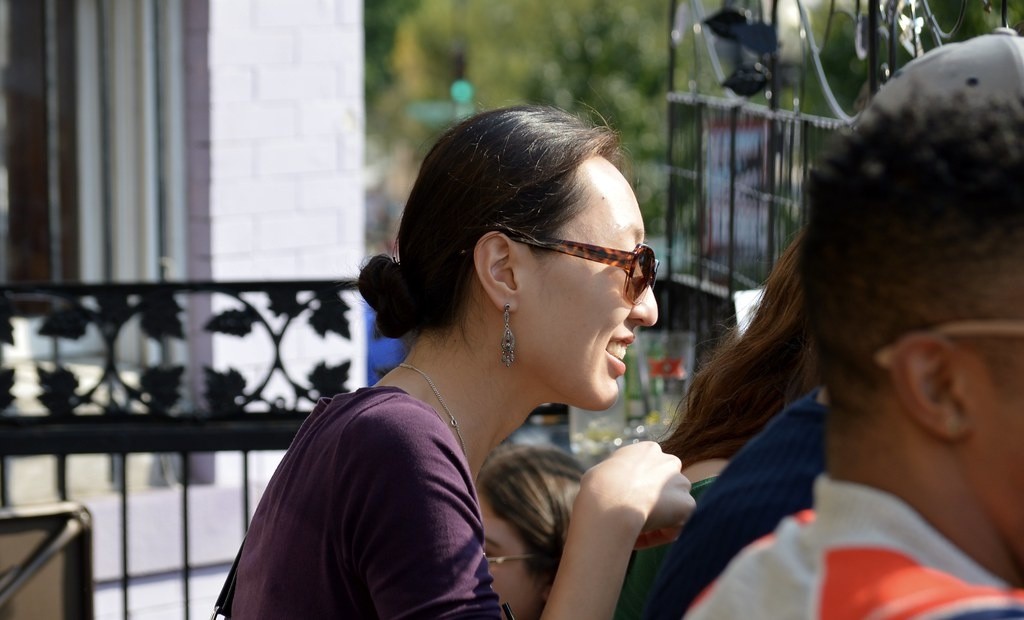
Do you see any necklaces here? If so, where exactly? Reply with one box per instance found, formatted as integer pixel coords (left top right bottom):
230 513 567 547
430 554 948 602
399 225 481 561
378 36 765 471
399 362 467 463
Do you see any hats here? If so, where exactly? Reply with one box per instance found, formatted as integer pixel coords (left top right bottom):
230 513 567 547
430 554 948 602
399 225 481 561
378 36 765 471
837 27 1024 147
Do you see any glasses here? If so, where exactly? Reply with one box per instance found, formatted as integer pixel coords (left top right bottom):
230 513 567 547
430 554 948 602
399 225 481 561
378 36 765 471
509 236 660 306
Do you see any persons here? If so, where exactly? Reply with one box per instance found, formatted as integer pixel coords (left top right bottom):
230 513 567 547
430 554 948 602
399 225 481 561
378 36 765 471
233 105 697 620
476 28 1023 620
